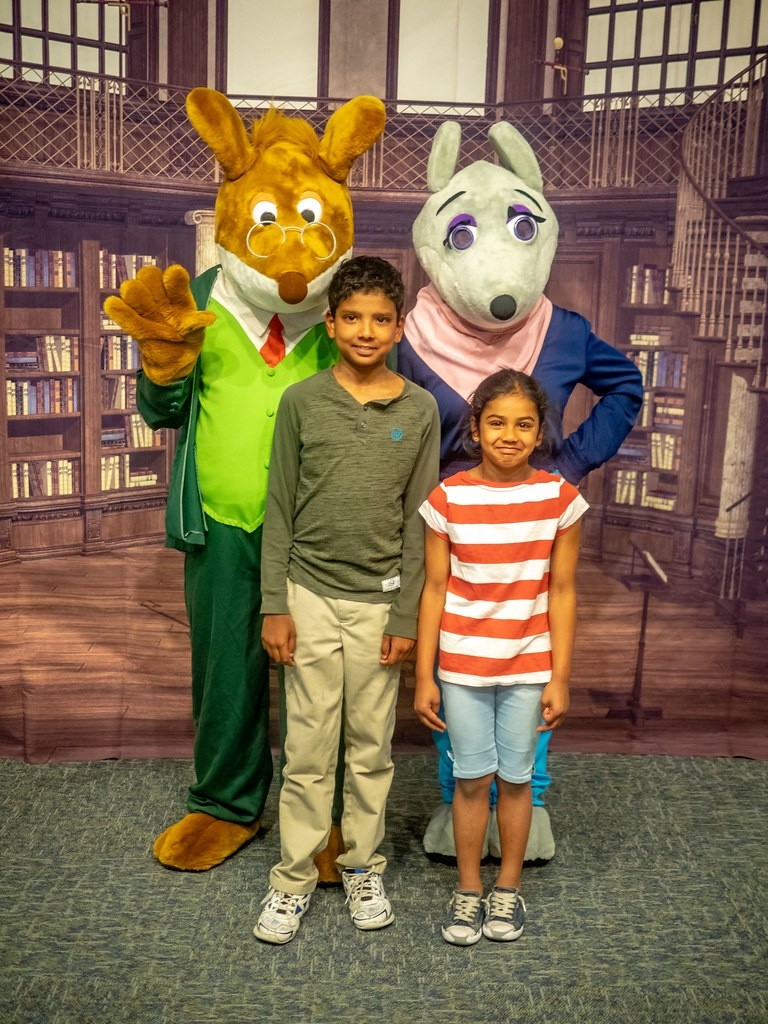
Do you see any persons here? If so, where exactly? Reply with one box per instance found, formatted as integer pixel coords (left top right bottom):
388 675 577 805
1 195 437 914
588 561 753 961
253 255 441 945
413 368 591 946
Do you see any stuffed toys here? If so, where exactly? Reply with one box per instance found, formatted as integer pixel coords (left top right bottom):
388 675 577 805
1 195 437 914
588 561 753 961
397 120 644 868
103 87 391 884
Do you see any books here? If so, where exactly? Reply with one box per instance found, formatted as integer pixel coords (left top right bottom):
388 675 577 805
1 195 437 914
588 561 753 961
100 334 142 370
101 374 139 409
642 391 686 431
6 377 79 416
9 458 81 499
99 247 166 289
625 350 689 390
100 453 158 492
100 413 166 449
4 335 79 373
629 324 673 346
617 431 683 472
609 469 678 512
100 305 124 330
4 247 76 288
623 263 676 306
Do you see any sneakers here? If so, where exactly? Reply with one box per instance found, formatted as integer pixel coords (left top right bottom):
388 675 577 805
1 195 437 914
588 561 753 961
441 888 483 945
253 884 311 944
483 884 527 941
342 865 395 929
